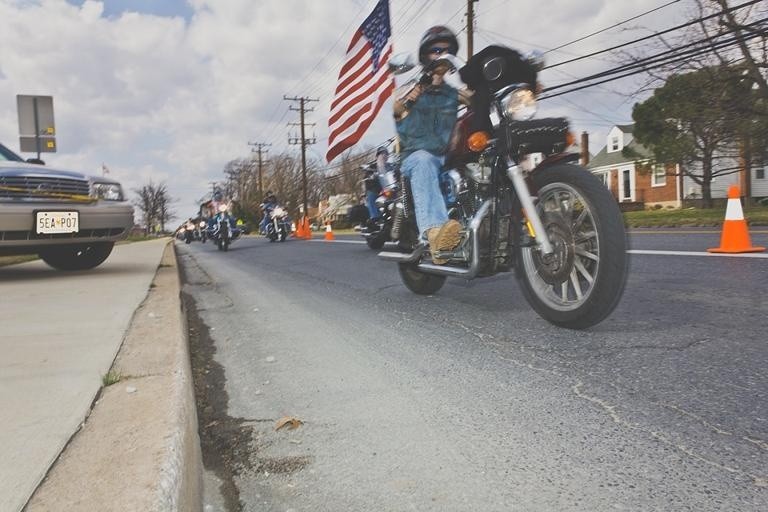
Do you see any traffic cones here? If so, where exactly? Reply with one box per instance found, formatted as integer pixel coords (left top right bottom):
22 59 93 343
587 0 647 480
296 221 304 238
705 183 768 254
324 219 336 240
303 216 311 240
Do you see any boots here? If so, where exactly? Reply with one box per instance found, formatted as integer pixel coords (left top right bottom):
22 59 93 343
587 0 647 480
425 219 463 266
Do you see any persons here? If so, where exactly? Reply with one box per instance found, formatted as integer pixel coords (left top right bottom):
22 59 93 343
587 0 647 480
258 190 283 229
364 146 395 225
392 26 543 265
207 188 238 234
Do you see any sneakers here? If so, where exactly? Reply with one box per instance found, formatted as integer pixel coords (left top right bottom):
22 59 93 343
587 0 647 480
366 217 379 231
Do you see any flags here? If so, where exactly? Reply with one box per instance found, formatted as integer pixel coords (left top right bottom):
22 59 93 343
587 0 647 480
326 0 397 164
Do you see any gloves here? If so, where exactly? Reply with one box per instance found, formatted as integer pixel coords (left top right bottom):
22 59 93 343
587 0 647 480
434 54 466 91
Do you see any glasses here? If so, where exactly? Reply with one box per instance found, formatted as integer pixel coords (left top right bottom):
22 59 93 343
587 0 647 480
424 47 449 55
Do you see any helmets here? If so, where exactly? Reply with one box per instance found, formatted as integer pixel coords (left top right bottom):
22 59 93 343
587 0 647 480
418 24 459 72
375 145 388 157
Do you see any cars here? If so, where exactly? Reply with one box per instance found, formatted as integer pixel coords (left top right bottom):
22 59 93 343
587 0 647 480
0 142 136 271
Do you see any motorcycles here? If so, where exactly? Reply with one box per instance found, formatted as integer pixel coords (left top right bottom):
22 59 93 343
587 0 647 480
257 200 291 241
176 203 250 253
353 163 408 252
377 43 630 333
